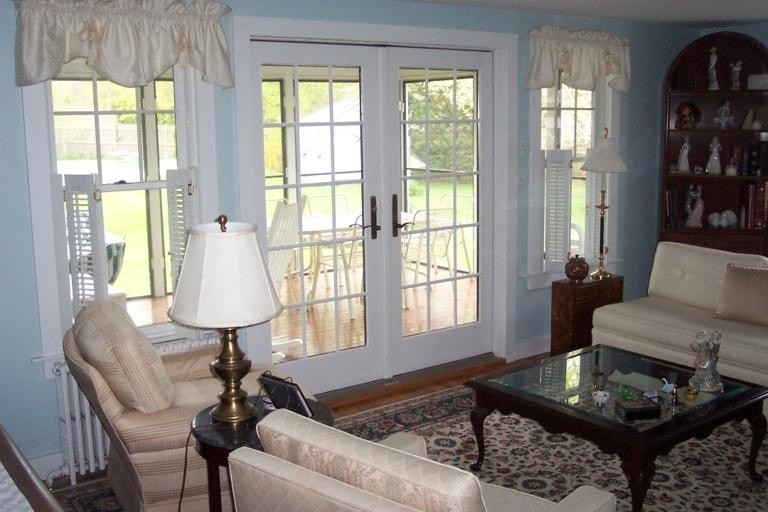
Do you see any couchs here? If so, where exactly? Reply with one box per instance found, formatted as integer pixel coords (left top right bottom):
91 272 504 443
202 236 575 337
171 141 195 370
592 242 768 417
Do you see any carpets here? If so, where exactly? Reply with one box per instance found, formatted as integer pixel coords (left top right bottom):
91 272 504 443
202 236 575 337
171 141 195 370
53 384 768 512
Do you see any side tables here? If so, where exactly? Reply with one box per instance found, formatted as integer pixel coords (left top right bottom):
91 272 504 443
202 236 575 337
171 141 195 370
191 395 333 512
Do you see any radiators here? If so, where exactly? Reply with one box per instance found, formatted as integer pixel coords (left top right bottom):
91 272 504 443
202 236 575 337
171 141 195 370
46 337 222 488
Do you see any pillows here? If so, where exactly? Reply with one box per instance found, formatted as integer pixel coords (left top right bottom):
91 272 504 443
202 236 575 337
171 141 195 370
714 263 768 327
74 299 174 414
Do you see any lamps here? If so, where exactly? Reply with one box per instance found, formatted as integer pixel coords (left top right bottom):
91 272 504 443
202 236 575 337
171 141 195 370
168 215 283 423
580 127 629 279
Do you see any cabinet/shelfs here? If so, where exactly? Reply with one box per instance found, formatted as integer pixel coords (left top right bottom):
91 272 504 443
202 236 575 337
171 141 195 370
659 31 768 257
551 274 623 356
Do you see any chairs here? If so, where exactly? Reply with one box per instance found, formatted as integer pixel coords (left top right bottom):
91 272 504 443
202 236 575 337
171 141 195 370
63 328 317 512
266 194 473 320
228 407 616 512
